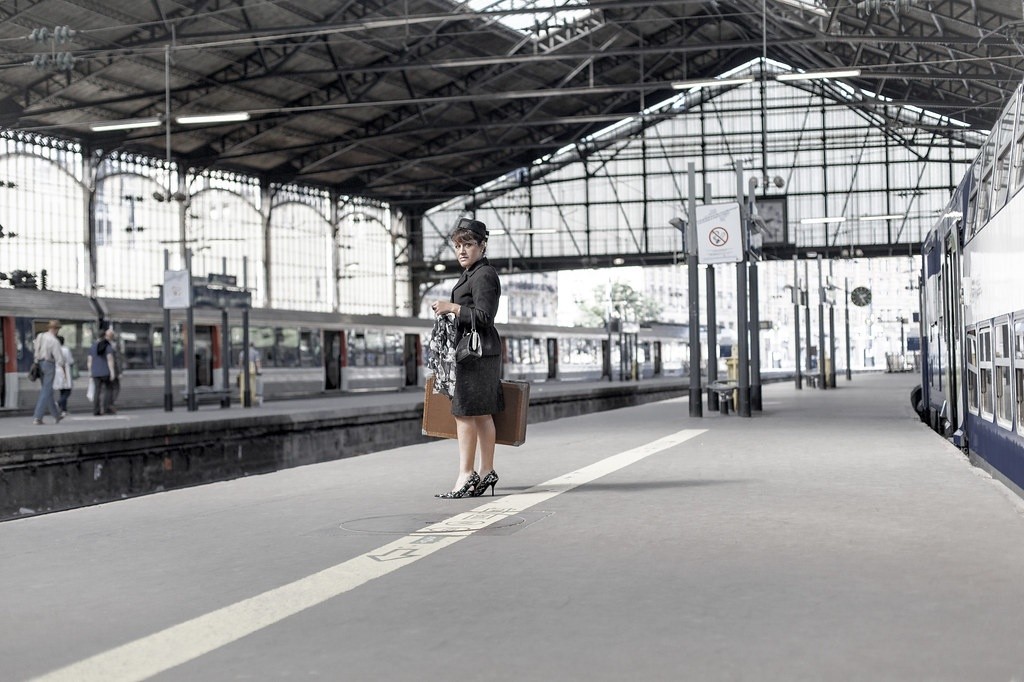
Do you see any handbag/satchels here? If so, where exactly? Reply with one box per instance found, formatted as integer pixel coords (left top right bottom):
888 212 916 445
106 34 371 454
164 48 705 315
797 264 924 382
28 363 40 382
236 375 242 388
455 307 482 365
86 377 96 402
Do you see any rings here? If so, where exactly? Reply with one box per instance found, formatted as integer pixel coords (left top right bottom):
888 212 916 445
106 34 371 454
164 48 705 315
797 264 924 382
432 305 436 310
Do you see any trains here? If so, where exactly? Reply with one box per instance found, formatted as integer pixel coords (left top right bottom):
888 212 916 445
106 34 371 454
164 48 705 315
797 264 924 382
918 83 1024 498
0 270 738 416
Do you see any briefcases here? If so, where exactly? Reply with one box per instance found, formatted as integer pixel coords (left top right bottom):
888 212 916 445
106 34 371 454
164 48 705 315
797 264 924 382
421 372 530 447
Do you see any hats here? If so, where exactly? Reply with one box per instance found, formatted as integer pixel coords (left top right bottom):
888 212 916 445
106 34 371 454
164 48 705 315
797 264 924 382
97 329 105 338
458 217 486 238
46 320 62 328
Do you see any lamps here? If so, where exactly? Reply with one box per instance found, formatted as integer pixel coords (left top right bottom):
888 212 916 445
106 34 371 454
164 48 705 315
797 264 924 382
176 112 251 124
90 116 162 131
672 75 756 89
775 68 863 80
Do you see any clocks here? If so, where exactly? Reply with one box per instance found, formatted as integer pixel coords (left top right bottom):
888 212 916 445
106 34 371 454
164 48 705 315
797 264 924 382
740 196 788 248
851 286 872 306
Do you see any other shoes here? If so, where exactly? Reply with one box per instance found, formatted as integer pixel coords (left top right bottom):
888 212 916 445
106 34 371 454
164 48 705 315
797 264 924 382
55 412 65 424
33 418 45 424
103 409 116 415
93 412 103 415
55 402 61 412
61 411 70 416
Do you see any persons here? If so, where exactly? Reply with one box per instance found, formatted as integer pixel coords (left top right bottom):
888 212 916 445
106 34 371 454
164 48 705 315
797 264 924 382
52 335 74 412
31 319 65 424
431 219 503 499
104 329 123 411
87 330 116 415
237 342 264 406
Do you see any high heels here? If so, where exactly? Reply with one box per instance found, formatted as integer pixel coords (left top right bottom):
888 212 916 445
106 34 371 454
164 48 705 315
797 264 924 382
462 470 499 496
434 471 480 499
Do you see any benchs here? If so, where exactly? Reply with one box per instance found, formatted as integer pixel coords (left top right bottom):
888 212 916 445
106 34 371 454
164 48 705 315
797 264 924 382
706 379 737 414
181 386 236 404
803 368 820 387
